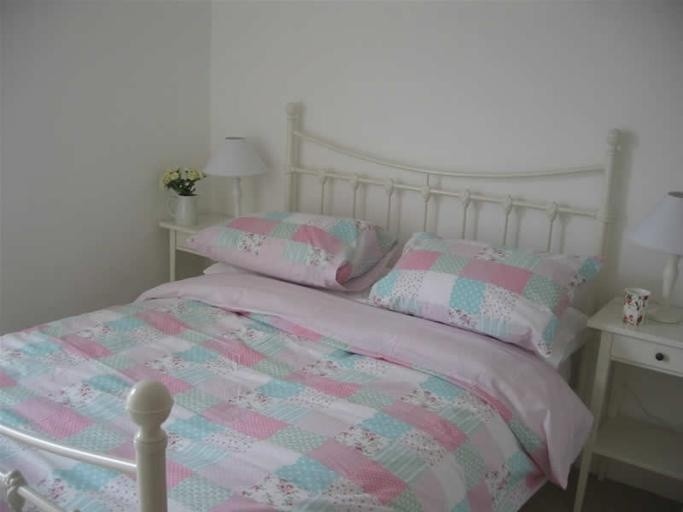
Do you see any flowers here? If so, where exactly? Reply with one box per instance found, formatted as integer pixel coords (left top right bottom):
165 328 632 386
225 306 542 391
159 165 207 196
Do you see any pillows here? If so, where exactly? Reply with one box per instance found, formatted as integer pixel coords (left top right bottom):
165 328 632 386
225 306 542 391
368 230 608 359
175 210 398 293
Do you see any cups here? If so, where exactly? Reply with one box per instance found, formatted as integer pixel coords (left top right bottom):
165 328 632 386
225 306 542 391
622 287 651 330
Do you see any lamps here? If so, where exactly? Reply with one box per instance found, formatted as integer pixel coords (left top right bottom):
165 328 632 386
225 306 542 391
202 137 268 219
623 191 683 325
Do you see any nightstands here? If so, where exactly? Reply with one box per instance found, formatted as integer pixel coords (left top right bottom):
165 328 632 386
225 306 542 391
158 213 235 282
573 295 683 512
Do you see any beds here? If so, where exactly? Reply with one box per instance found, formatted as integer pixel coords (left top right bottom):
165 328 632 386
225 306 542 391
0 101 623 512
